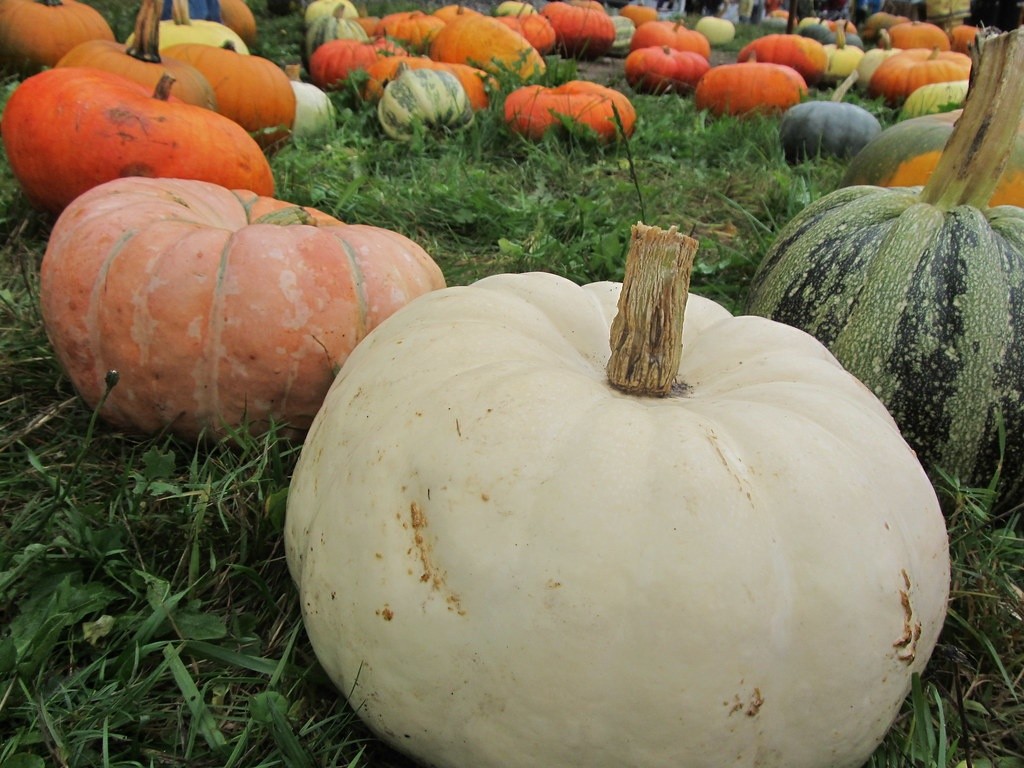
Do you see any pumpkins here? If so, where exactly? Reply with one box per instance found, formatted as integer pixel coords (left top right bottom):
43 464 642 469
37 176 446 431
305 0 986 163
284 219 949 768
0 1 337 208
733 20 1024 519
